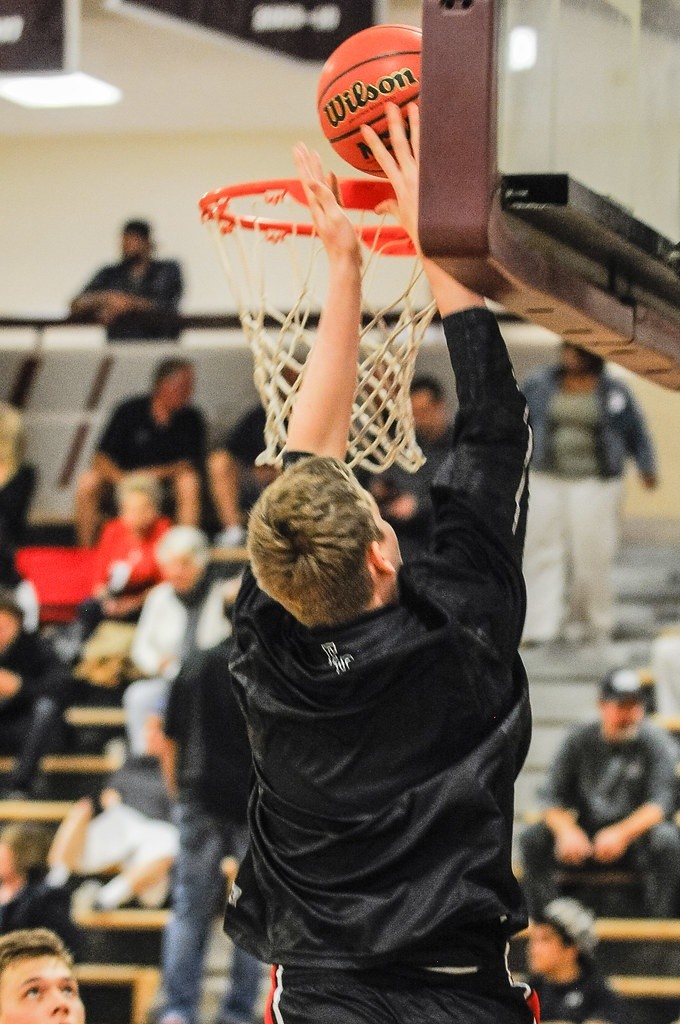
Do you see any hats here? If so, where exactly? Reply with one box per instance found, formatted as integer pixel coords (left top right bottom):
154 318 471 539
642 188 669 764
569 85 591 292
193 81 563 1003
599 669 645 698
531 896 599 968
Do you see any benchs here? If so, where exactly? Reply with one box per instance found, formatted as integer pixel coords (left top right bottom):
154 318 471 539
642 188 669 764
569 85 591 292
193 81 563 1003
0 502 680 1024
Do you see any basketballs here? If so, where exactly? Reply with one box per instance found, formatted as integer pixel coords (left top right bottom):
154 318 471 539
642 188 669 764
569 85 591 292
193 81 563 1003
314 25 422 177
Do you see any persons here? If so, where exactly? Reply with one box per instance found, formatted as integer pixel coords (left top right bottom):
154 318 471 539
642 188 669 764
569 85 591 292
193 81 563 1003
227 101 540 1024
519 893 622 1024
48 357 448 1024
69 220 184 343
519 666 680 916
519 334 657 643
1 595 68 799
1 820 69 935
1 400 40 587
0 930 96 1024
